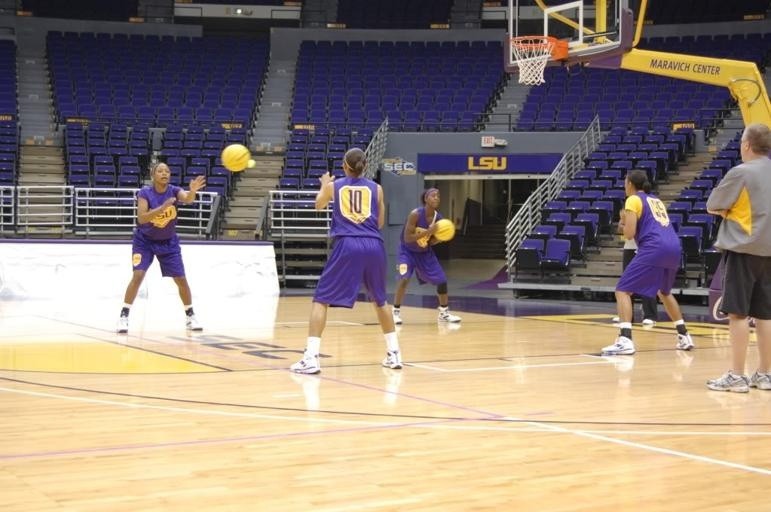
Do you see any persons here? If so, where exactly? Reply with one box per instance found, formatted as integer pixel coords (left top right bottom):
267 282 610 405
391 188 461 324
705 123 771 393
596 170 695 355
117 161 206 333
288 148 402 373
613 205 657 325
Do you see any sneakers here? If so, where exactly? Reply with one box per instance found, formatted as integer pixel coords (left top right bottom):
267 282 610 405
642 318 658 324
383 350 403 369
185 309 202 330
749 371 771 389
707 371 748 392
118 307 129 334
393 307 402 324
290 349 321 374
676 330 694 350
438 307 461 322
613 316 622 322
601 334 635 354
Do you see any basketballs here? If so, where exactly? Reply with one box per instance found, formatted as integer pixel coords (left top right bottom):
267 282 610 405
221 144 250 173
434 218 456 241
